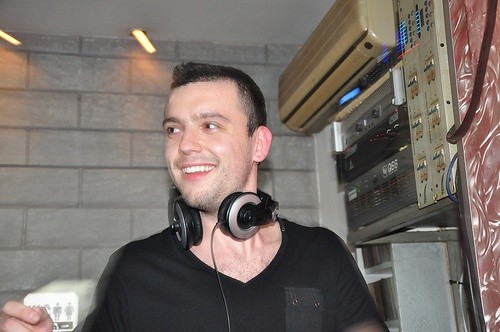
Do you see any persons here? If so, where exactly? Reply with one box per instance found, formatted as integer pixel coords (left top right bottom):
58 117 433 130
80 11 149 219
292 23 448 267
0 62 389 332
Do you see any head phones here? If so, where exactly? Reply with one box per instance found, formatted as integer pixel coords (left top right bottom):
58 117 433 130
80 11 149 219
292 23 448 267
168 183 279 251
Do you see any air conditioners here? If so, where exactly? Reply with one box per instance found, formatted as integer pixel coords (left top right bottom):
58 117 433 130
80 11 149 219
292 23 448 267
278 0 398 135
